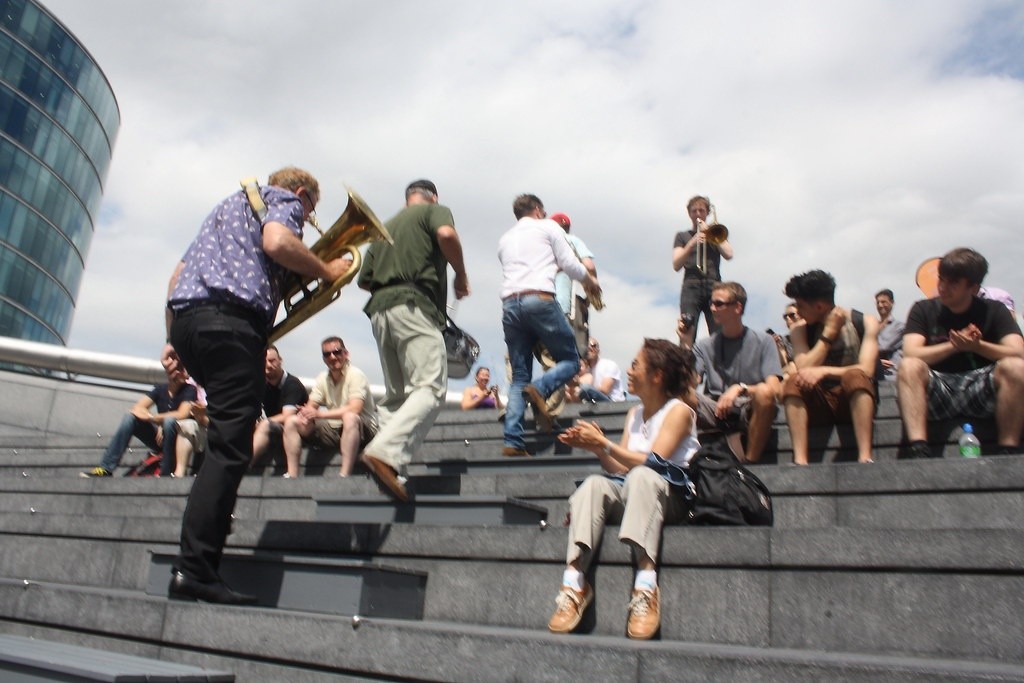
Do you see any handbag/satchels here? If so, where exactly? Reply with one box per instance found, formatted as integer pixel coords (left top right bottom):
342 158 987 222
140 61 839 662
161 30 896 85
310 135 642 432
684 445 774 528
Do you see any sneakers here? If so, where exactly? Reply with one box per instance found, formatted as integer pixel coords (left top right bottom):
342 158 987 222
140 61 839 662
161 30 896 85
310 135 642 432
628 587 660 639
79 467 113 479
547 580 594 632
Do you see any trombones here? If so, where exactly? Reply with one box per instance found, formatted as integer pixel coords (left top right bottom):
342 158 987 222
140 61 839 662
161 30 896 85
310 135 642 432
696 203 729 274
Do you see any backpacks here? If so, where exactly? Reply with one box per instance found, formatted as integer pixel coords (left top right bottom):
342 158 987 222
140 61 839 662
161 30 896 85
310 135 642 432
124 455 163 478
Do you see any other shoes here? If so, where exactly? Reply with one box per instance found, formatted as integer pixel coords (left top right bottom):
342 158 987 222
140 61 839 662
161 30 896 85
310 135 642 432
995 443 1022 456
522 388 552 432
906 440 934 460
359 452 409 502
502 446 530 457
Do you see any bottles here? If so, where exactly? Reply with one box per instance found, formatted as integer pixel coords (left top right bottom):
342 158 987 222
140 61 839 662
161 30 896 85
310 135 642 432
959 423 981 458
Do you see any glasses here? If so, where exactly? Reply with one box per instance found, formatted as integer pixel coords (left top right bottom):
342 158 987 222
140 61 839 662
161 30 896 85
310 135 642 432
324 347 344 358
783 312 798 319
708 299 739 308
304 190 316 220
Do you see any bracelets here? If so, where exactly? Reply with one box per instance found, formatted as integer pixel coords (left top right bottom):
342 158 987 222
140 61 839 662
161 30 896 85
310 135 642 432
603 441 614 453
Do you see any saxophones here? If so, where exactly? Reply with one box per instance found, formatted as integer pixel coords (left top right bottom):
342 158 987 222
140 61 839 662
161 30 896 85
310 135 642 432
561 236 604 312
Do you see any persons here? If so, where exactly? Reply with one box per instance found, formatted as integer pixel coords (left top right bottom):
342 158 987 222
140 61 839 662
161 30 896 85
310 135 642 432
357 179 470 505
78 337 379 478
461 195 1024 464
547 336 700 637
161 166 352 604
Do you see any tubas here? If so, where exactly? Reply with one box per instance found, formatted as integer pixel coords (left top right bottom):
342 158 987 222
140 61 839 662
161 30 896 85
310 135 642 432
270 183 393 348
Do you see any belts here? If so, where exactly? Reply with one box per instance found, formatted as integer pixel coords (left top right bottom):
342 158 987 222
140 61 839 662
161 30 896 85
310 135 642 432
503 290 555 302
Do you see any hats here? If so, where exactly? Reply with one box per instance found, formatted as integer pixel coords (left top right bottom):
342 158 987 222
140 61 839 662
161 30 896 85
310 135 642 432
407 181 438 197
552 214 571 228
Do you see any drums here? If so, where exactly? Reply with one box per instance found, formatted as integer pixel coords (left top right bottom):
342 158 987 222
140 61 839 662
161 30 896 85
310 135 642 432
441 326 480 379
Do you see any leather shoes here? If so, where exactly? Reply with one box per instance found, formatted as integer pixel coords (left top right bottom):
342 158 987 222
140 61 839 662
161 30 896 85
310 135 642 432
168 572 260 604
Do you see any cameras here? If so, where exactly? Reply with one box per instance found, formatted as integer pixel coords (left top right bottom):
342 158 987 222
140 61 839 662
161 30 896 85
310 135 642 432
682 312 695 328
